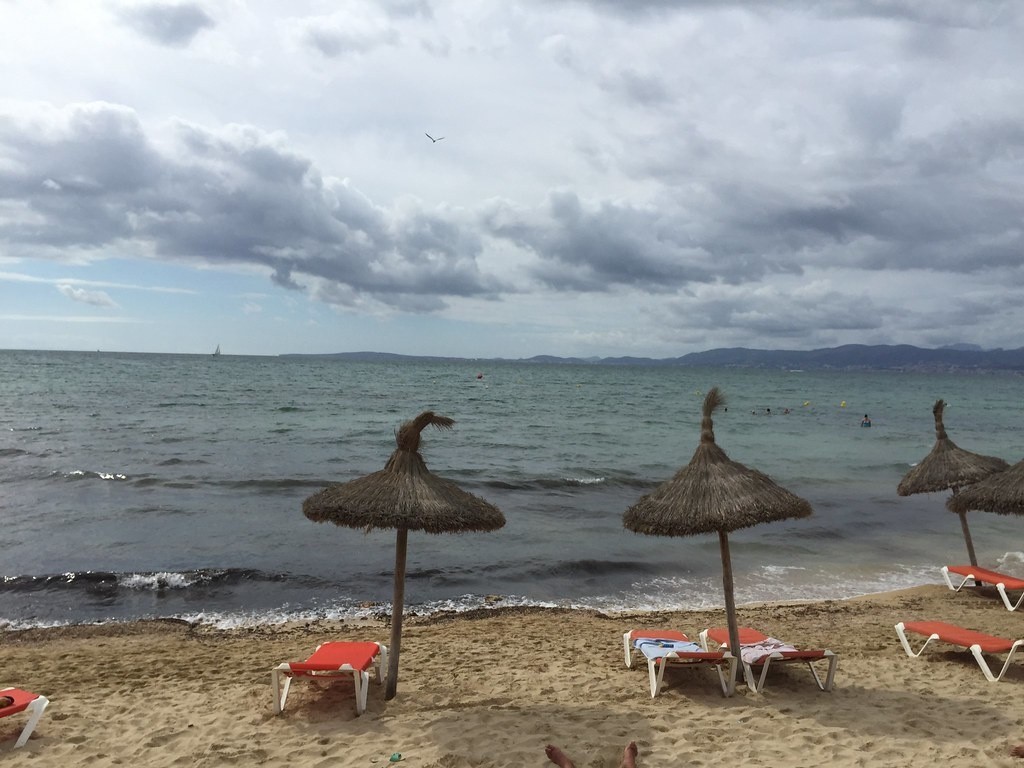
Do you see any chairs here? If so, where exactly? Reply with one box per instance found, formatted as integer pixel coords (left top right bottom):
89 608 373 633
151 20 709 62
941 565 1024 612
0 687 49 749
894 620 1024 682
700 628 838 695
271 642 386 716
623 629 728 699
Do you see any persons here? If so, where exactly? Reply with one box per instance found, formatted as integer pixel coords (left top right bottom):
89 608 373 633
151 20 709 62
545 741 639 768
1010 745 1024 758
0 696 14 708
725 409 791 415
861 414 871 427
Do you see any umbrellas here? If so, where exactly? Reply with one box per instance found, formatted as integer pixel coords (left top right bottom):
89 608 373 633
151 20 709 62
622 388 811 682
898 400 1024 587
302 413 505 701
